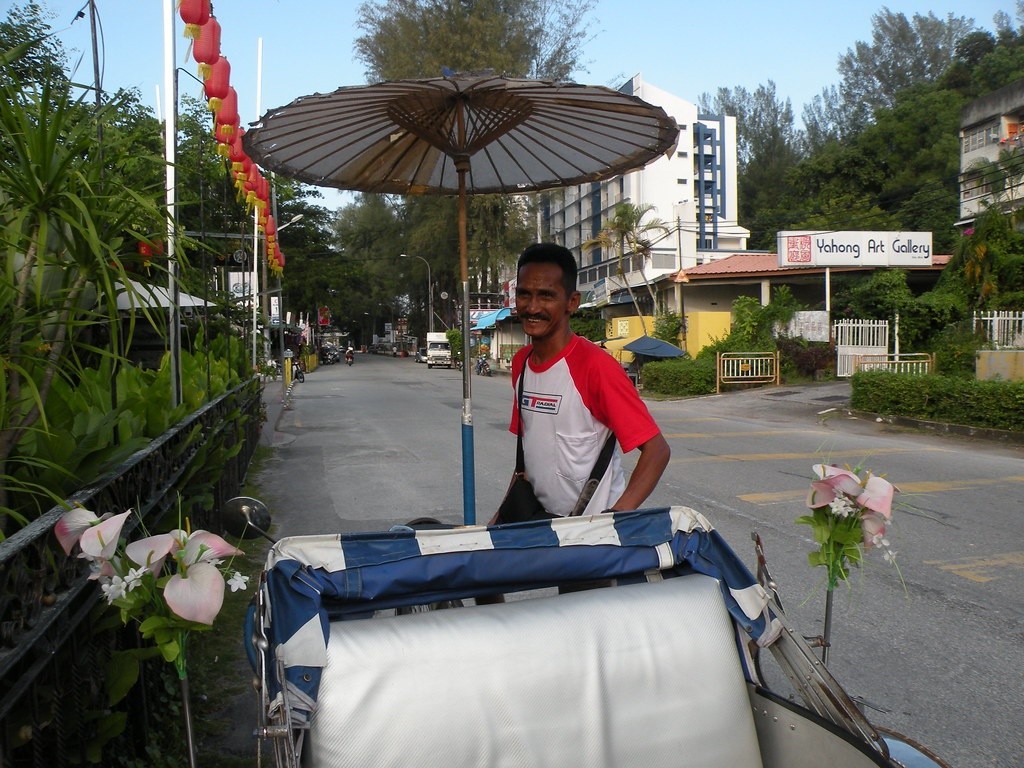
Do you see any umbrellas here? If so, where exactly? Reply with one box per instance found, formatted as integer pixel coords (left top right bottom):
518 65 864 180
241 72 682 533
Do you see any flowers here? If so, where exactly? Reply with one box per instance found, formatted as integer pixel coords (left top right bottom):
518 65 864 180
42 487 255 680
798 437 922 590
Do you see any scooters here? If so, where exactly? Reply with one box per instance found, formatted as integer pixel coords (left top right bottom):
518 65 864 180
346 351 354 366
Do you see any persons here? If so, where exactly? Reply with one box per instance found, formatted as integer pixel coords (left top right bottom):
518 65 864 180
487 243 672 528
346 347 355 362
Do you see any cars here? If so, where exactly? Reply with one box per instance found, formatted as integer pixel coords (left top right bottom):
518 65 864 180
415 348 427 363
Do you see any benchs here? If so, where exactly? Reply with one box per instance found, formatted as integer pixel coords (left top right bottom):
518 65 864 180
302 569 768 768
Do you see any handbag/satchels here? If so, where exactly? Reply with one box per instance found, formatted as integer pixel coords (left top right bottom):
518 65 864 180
493 473 564 526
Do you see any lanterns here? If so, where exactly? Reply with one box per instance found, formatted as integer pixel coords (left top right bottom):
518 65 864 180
179 0 287 278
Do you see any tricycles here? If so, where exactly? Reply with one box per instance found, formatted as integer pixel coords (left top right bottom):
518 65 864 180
217 495 901 767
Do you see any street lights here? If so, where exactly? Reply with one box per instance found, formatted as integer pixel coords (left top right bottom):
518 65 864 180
400 254 434 332
262 204 304 374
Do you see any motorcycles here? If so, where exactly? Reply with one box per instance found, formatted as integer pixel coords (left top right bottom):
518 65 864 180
476 354 492 376
453 352 463 371
277 361 305 383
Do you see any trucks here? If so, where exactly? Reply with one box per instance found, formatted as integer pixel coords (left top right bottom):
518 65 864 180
426 331 452 369
